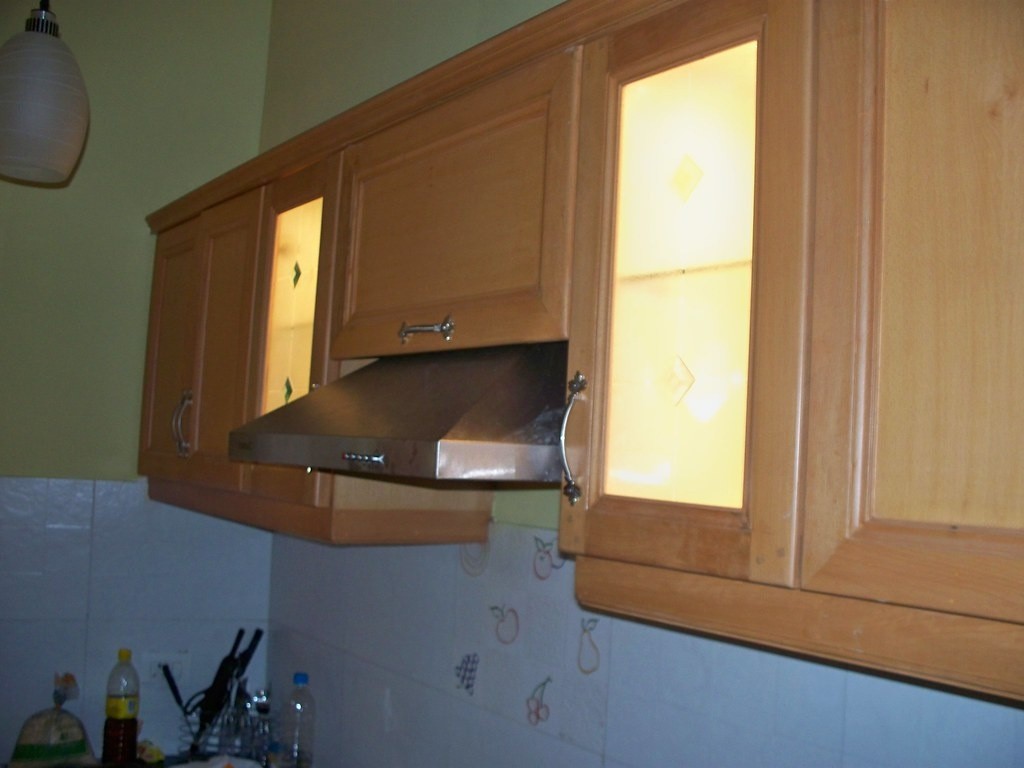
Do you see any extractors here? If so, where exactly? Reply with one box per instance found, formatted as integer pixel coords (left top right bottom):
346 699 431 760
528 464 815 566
227 341 570 484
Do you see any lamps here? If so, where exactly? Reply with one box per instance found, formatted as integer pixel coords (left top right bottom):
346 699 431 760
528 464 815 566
0 0 89 184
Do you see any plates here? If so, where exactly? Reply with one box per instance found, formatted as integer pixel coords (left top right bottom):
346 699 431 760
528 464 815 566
172 756 262 768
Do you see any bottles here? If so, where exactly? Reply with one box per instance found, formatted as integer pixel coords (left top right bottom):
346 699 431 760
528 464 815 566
101 648 139 766
282 671 313 768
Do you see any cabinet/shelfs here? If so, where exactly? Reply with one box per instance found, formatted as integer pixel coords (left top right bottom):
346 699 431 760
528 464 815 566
142 1 1024 706
